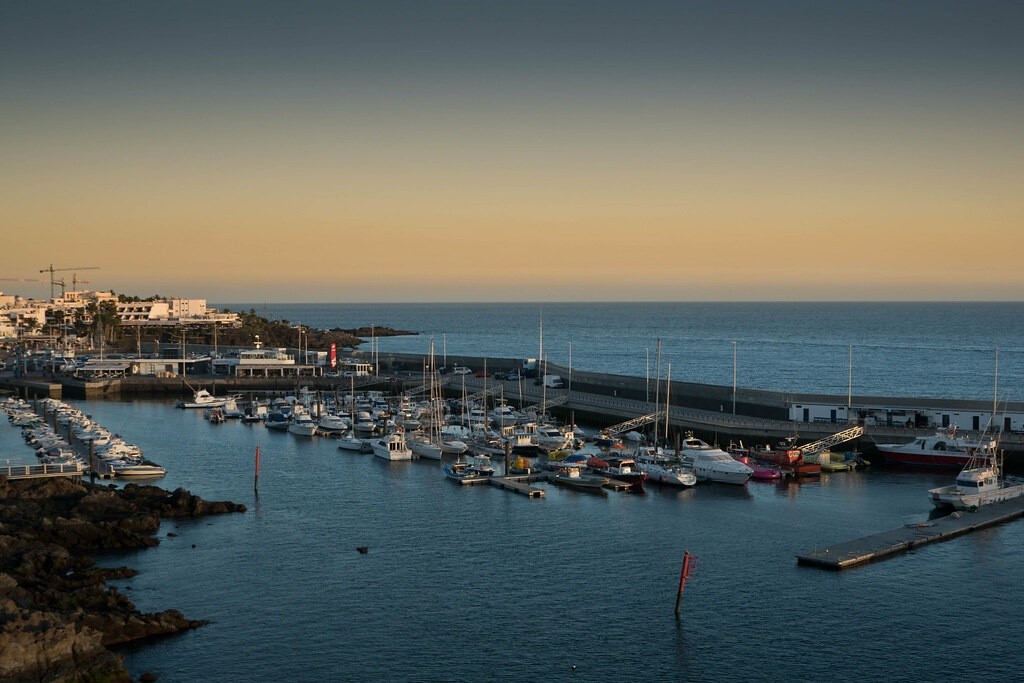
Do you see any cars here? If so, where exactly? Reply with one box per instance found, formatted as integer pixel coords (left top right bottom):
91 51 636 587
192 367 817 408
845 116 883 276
495 371 509 380
534 377 545 386
474 372 492 378
506 373 523 381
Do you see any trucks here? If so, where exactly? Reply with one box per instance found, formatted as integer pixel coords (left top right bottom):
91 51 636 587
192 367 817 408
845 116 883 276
543 374 564 389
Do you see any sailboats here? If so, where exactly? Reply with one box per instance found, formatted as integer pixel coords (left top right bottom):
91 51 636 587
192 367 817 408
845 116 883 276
922 346 1022 509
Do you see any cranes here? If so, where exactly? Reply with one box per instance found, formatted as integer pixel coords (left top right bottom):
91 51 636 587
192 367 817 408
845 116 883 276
40 263 103 300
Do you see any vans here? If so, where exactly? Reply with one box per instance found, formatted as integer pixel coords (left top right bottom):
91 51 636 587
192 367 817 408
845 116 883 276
452 366 474 376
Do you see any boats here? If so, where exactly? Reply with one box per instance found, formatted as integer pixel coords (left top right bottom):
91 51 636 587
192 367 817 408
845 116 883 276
1 391 164 478
873 420 1000 467
177 327 868 488
